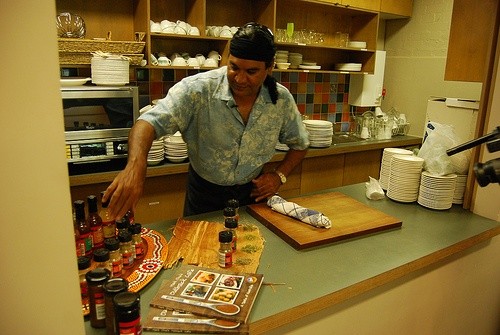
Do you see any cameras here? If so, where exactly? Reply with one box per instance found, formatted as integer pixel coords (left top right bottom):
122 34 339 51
474 157 500 187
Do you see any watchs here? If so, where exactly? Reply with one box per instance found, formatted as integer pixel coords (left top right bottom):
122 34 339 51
274 170 287 185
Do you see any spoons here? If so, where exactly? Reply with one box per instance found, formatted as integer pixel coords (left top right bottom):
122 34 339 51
160 294 240 315
153 317 241 329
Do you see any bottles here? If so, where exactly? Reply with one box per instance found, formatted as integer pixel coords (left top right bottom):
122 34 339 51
71 186 147 335
217 231 233 268
223 207 237 231
227 199 239 228
223 217 238 252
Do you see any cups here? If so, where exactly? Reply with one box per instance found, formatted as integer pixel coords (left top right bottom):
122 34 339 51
147 17 200 35
275 22 349 49
139 104 155 116
353 108 407 141
206 26 242 38
149 50 222 68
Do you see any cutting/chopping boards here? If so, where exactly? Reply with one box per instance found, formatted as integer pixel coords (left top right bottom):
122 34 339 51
246 191 404 249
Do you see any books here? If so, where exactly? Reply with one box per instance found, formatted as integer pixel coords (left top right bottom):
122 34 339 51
140 266 265 335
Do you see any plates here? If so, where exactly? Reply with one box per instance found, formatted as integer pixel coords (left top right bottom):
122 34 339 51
377 146 468 210
273 50 364 72
274 116 334 150
350 41 367 48
152 98 164 104
59 55 131 86
146 130 189 166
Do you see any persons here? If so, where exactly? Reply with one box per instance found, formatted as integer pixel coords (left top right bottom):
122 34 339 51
101 22 309 220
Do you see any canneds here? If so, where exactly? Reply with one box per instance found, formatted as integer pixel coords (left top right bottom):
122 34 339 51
85 268 142 335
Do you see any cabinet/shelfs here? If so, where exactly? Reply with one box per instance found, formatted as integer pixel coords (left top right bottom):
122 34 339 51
134 0 380 75
300 149 380 195
